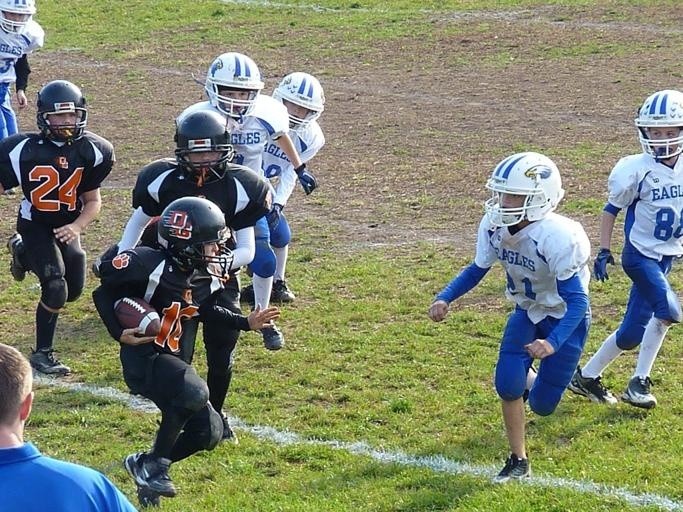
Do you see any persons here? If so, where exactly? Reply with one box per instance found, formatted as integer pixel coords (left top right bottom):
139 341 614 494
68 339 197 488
92 52 328 509
1 80 116 377
0 0 47 203
566 87 683 410
0 345 135 512
426 149 594 488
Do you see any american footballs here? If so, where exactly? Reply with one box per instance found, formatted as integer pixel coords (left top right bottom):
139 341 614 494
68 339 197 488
113 297 161 338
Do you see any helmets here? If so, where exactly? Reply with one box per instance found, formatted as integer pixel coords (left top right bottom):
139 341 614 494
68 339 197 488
484 151 565 228
156 196 237 281
173 109 236 187
270 71 326 134
35 78 88 143
0 0 35 34
205 51 265 118
635 89 683 160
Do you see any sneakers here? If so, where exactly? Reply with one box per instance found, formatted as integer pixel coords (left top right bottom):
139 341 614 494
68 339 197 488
270 279 296 303
494 452 532 484
6 232 26 282
124 452 177 510
29 348 72 375
220 408 240 446
522 363 538 404
620 376 658 409
567 365 619 406
259 320 285 350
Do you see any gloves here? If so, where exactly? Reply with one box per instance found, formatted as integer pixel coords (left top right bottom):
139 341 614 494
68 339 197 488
293 162 317 195
594 248 615 282
